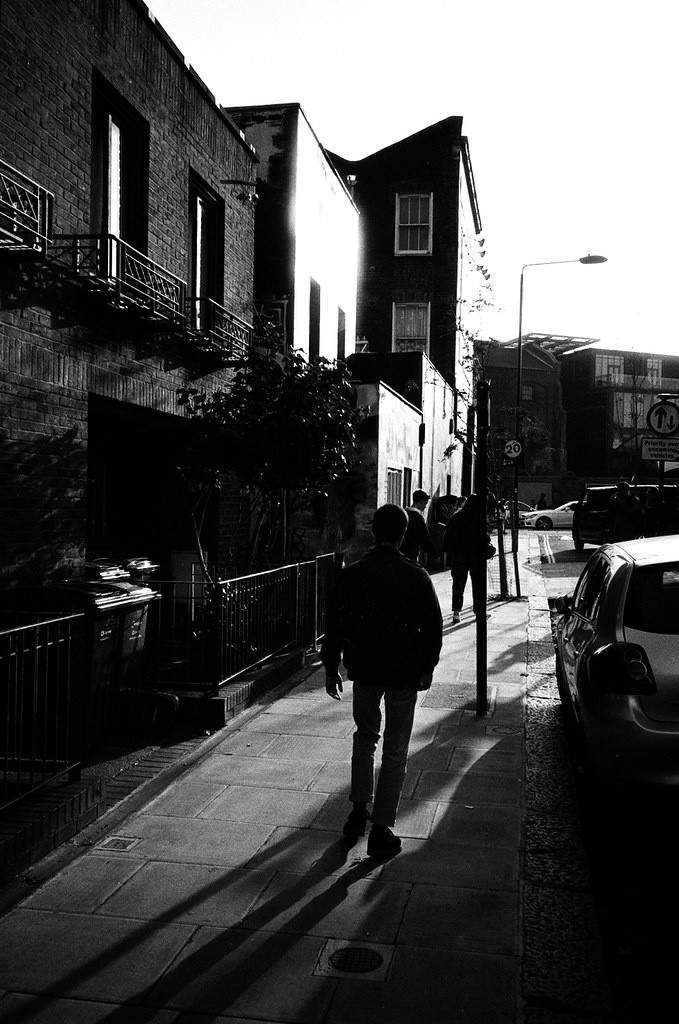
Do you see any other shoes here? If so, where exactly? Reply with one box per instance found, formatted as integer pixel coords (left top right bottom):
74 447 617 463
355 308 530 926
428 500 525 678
453 614 460 622
366 829 402 856
344 808 370 835
485 614 491 620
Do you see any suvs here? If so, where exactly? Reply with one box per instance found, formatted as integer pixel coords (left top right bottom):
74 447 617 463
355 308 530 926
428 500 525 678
573 485 638 551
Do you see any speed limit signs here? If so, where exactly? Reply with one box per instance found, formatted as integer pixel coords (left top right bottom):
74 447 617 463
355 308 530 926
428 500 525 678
503 440 522 460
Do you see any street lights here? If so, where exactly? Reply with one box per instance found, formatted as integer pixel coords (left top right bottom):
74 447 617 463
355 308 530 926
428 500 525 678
505 256 610 557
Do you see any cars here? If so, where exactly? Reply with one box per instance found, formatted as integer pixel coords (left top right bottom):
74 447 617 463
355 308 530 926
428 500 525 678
554 535 679 796
501 500 538 523
519 501 589 530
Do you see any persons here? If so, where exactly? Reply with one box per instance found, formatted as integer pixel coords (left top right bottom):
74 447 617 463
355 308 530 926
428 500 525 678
608 480 663 549
318 504 444 859
399 489 443 568
440 494 493 623
537 493 547 510
499 496 512 534
441 496 467 572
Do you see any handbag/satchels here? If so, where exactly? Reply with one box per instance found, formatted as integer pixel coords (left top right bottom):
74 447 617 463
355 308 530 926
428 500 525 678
486 544 496 559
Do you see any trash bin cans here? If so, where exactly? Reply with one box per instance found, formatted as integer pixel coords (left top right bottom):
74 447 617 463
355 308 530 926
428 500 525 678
52 573 163 769
